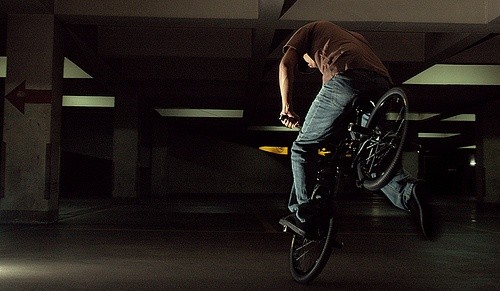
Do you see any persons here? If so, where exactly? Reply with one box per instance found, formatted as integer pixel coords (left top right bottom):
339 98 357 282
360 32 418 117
278 19 441 241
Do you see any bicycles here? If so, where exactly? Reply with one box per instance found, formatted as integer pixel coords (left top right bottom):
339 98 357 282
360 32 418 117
278 86 410 283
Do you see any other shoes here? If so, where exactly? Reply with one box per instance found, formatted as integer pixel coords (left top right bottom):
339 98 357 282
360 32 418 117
412 179 442 240
278 211 316 239
